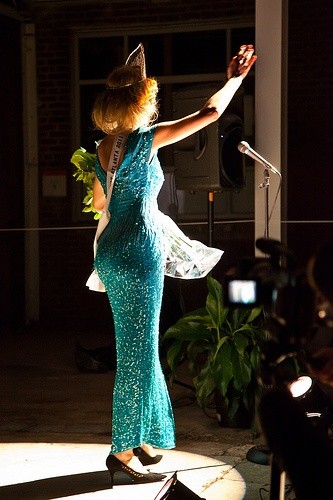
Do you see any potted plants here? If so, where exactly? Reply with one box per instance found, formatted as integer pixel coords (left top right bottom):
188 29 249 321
162 275 268 429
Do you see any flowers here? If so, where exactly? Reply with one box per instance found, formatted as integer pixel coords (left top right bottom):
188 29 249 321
70 146 192 262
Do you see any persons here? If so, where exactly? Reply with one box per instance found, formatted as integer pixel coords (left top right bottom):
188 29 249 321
92 46 258 486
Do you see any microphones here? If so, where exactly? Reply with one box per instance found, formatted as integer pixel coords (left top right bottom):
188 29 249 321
237 140 281 176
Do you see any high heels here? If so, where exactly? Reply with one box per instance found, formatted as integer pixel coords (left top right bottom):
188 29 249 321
106 454 167 489
132 447 163 467
